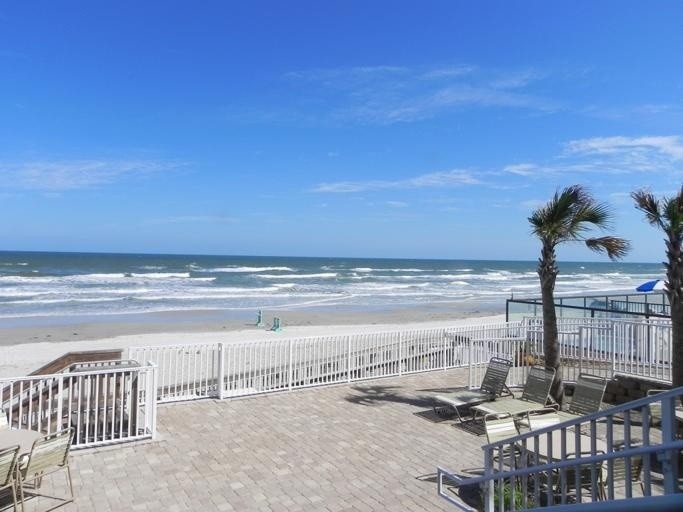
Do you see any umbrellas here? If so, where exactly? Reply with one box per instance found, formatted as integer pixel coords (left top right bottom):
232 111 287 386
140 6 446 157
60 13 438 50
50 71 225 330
634 278 670 314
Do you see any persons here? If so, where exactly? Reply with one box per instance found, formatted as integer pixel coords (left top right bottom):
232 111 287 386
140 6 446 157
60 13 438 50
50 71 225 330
453 338 468 365
406 345 418 371
419 353 432 370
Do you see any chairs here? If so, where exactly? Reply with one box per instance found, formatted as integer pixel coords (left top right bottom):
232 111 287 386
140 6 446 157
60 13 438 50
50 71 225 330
417 356 683 504
0 427 75 512
